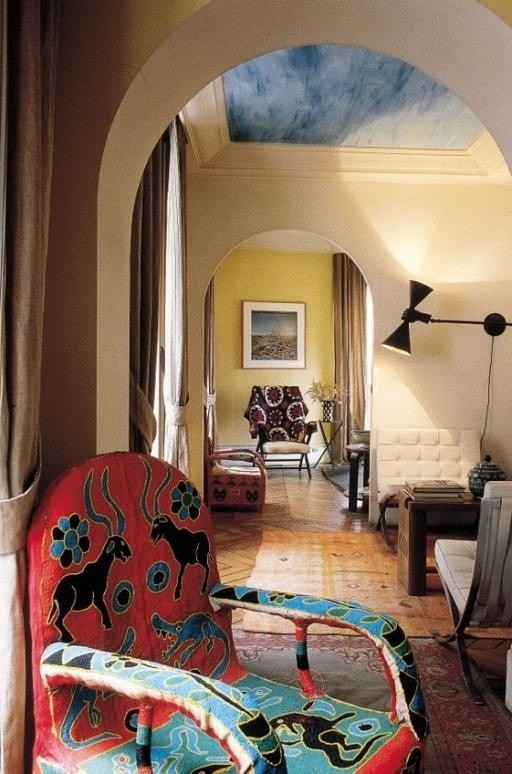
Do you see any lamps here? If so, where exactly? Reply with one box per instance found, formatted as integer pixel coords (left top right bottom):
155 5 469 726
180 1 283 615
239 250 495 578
380 278 512 357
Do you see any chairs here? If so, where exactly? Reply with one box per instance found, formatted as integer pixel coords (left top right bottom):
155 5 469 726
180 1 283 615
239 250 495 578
205 436 266 515
428 482 512 707
245 385 315 480
28 451 433 774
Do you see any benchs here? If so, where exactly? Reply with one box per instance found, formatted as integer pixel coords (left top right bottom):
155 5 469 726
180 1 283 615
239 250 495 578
372 428 482 546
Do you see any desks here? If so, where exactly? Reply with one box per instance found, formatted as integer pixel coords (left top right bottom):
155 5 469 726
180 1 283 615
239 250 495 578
393 487 482 596
312 419 344 470
343 443 371 513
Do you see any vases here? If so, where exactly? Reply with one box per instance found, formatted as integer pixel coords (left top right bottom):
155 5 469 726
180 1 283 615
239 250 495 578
350 430 370 443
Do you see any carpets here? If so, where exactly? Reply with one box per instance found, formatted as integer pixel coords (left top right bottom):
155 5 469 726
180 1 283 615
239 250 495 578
236 626 512 774
319 458 365 490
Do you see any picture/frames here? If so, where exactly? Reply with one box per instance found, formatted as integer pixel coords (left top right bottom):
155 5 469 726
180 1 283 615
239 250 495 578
242 300 307 372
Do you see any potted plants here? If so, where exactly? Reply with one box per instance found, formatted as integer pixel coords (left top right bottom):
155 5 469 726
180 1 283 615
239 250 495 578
307 378 349 422
